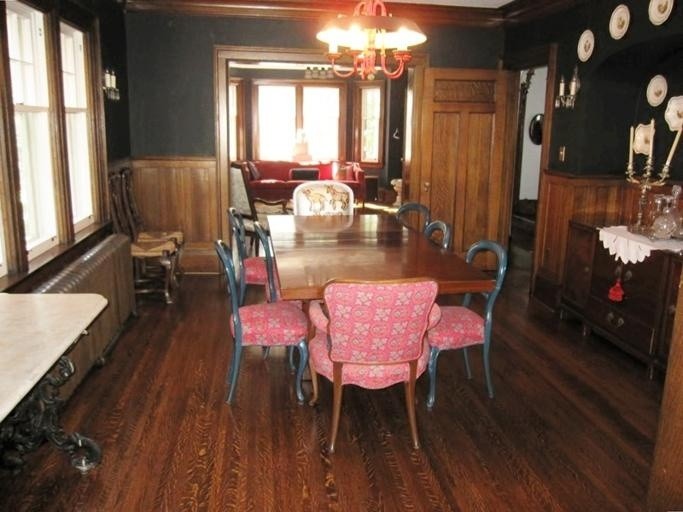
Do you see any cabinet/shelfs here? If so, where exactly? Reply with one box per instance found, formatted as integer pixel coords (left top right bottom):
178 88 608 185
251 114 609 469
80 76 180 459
562 217 681 383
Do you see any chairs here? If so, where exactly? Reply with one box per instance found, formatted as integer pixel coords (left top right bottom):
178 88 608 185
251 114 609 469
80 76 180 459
227 209 274 305
395 203 427 228
293 179 354 217
421 220 450 250
422 241 506 409
250 228 310 358
214 241 310 407
305 269 439 458
122 168 184 291
106 169 178 306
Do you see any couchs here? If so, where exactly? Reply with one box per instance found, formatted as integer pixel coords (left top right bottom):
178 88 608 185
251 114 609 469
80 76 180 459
237 160 364 213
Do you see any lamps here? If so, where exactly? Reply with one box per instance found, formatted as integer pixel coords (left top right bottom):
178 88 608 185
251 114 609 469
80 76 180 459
313 3 427 82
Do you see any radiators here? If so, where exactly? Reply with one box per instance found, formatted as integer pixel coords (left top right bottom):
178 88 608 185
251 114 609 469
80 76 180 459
29 227 134 408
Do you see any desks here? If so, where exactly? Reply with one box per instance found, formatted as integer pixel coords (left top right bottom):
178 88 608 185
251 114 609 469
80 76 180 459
263 215 491 409
0 292 111 512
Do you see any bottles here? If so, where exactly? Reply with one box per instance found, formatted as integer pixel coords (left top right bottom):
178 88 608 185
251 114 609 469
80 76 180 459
648 185 683 239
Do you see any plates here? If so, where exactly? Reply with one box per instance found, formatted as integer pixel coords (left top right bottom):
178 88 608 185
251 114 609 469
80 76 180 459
645 74 668 108
633 123 656 156
648 0 675 26
608 3 631 41
664 94 682 134
576 28 596 62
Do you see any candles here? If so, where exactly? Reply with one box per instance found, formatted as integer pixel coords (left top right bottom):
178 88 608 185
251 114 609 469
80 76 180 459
666 126 682 165
648 116 655 157
627 124 634 163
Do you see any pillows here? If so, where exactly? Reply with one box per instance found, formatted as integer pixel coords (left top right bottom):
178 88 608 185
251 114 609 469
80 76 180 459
326 161 348 181
247 161 263 180
